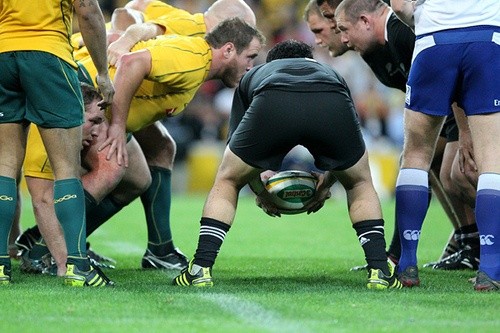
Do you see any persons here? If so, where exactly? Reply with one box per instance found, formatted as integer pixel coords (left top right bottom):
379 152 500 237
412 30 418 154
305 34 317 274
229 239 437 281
336 0 479 270
171 40 402 291
0 0 459 287
393 0 500 292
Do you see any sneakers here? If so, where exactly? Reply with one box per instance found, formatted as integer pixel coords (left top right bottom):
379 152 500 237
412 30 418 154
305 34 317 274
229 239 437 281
12 225 117 277
366 262 405 292
141 248 191 271
392 263 420 288
0 255 12 285
474 271 500 292
62 262 117 289
172 258 214 289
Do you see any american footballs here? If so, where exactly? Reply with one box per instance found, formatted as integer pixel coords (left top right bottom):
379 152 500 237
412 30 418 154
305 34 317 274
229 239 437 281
265 170 319 214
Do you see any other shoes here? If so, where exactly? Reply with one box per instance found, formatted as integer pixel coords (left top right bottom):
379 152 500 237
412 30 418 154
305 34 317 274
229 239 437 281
421 248 480 271
349 252 401 273
